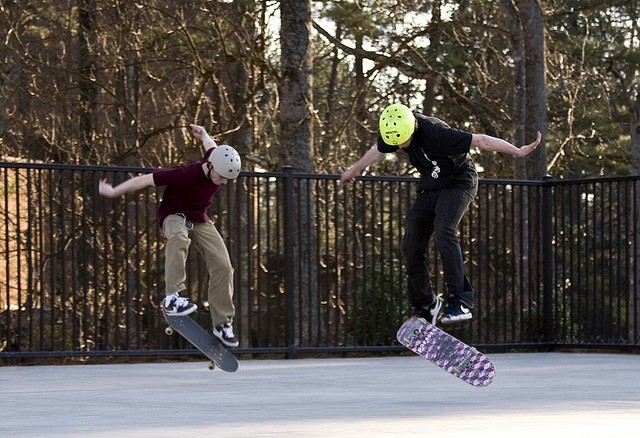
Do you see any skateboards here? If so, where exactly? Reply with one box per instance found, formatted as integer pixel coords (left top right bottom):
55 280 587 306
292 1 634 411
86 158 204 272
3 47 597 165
397 316 496 387
163 298 238 372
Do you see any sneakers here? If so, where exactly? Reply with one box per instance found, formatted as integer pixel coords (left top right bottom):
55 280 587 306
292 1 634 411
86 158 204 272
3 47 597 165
163 293 198 316
441 305 472 324
421 297 443 326
213 322 239 347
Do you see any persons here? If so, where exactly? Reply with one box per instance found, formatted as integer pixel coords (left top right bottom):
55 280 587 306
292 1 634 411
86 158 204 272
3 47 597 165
339 103 544 328
98 123 243 348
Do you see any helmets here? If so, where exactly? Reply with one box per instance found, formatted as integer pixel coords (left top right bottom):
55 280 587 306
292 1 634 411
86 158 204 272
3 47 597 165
209 145 241 179
379 104 415 146
